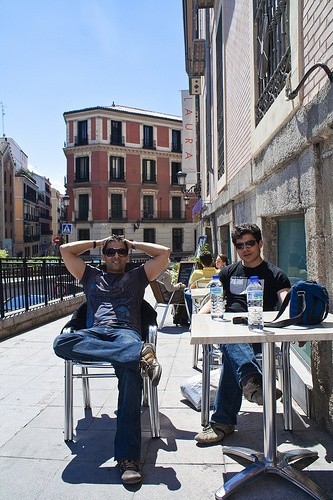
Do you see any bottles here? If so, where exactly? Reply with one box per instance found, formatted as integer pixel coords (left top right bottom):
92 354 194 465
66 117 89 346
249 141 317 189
210 275 224 322
246 276 264 333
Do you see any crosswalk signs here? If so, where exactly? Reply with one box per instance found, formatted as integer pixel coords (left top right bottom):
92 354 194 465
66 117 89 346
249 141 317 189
63 224 72 235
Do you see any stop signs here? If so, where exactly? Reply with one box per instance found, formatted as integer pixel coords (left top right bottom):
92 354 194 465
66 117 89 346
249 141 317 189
54 237 61 244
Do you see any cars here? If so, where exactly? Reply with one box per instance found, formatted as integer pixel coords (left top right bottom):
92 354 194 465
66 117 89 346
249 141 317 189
4 294 50 314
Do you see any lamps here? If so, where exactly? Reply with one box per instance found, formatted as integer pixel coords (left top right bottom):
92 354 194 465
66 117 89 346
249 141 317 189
177 170 196 206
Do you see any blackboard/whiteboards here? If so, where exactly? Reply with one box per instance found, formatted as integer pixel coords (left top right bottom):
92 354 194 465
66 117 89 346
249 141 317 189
177 262 195 287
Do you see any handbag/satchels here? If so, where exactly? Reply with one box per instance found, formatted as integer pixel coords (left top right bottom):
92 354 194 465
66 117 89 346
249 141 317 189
289 280 329 325
180 377 218 410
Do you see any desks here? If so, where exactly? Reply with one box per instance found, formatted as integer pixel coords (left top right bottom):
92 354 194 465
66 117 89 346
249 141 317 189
185 288 211 297
190 311 333 500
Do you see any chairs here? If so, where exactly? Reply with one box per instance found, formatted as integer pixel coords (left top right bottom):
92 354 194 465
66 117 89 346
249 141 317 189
60 298 160 443
149 280 190 330
183 278 294 432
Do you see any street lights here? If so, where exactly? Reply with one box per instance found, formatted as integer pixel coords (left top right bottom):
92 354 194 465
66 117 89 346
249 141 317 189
62 193 70 244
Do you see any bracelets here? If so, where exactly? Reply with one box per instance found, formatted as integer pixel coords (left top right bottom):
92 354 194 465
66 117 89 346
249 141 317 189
93 240 96 248
132 241 137 250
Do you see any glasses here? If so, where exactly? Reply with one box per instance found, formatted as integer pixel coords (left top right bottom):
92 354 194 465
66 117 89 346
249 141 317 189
235 240 256 250
103 248 128 258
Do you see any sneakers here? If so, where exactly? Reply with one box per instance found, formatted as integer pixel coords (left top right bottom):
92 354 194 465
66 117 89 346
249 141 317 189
140 343 162 387
242 376 282 406
195 420 235 443
116 457 141 483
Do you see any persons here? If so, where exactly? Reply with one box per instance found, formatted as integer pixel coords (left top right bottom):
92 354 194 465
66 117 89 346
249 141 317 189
53 235 172 484
216 254 229 271
155 257 190 327
188 222 292 444
189 251 221 304
